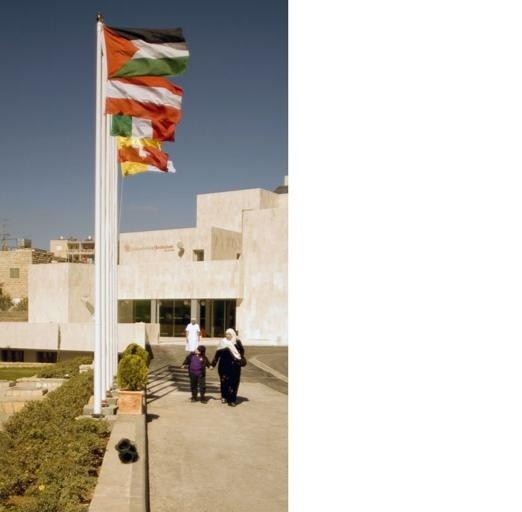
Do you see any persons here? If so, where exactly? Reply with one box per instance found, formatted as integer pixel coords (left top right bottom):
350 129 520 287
210 328 247 407
184 318 201 354
181 345 210 404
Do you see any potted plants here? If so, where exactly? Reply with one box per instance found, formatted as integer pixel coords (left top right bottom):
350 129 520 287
115 354 149 415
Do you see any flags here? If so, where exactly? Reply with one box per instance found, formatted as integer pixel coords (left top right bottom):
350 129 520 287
102 22 190 176
